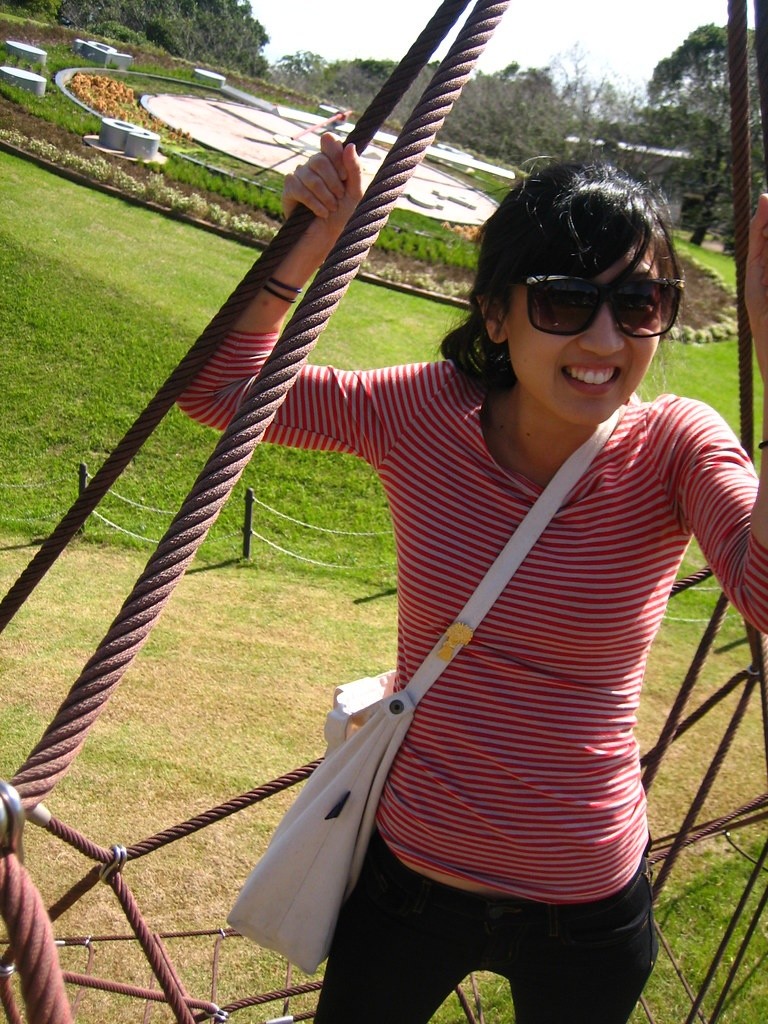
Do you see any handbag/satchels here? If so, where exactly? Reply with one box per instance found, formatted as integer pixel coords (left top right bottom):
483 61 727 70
227 671 415 976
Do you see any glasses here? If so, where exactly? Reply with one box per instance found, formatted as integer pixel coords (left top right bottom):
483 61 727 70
515 273 683 339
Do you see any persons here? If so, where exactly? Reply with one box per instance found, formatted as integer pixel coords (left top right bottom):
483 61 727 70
181 114 768 1024
